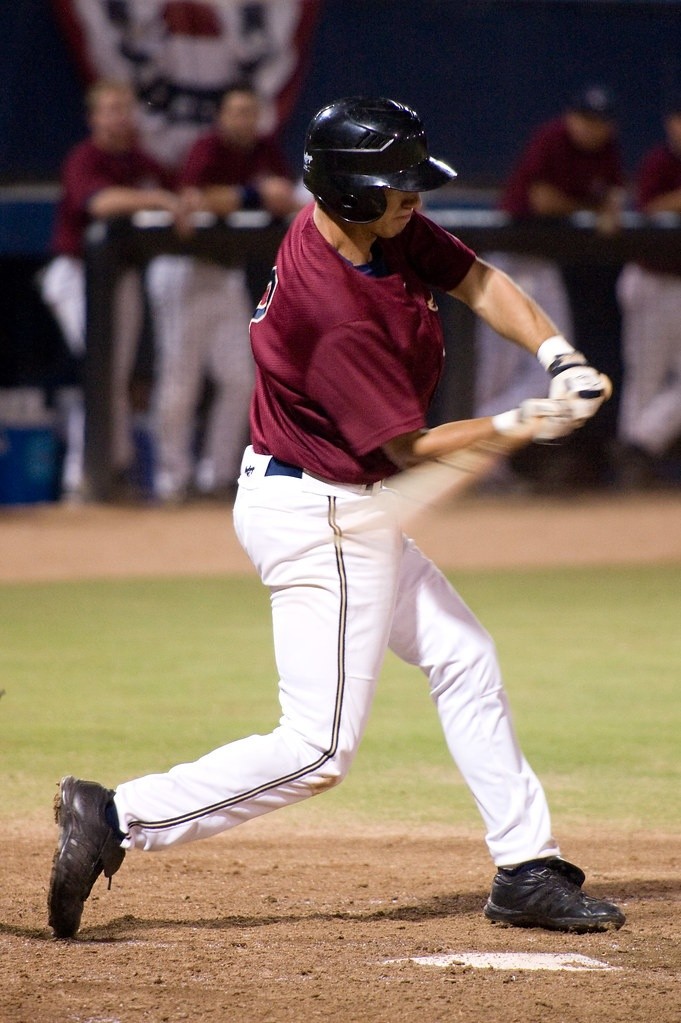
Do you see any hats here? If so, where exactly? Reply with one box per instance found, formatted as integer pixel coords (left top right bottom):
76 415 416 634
567 80 617 117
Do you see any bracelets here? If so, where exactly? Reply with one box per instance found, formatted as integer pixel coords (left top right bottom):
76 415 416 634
536 335 576 371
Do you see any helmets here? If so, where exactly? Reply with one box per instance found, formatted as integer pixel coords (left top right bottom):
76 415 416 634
301 95 458 224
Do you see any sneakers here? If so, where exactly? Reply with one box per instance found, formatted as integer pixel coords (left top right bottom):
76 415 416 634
46 775 126 939
484 856 626 932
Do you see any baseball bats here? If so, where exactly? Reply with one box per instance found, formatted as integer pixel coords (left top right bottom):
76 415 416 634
369 370 614 536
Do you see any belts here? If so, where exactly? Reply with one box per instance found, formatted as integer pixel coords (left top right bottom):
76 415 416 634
263 456 383 491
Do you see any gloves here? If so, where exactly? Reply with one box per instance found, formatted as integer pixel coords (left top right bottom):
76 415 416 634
516 350 612 440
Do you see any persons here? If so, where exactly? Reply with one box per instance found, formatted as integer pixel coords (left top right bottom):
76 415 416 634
474 87 626 424
148 87 292 504
617 104 681 462
49 74 191 500
44 97 626 939
